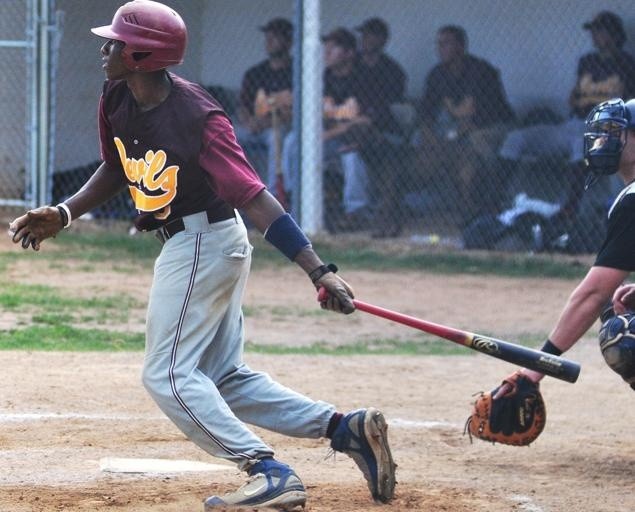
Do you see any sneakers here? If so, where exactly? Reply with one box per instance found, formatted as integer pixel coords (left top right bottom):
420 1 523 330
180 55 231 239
330 408 396 505
203 458 307 512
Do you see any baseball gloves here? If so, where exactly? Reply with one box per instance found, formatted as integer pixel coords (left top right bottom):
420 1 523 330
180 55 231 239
468 370 546 446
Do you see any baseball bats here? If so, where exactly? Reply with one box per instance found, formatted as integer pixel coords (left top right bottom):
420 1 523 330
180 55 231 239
317 287 581 382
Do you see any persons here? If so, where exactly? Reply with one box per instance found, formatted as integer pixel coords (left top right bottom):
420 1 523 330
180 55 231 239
229 12 634 240
8 0 399 510
490 97 635 402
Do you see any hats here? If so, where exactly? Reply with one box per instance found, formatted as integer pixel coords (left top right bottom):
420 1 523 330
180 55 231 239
256 17 296 42
582 10 625 32
319 27 357 50
355 17 390 42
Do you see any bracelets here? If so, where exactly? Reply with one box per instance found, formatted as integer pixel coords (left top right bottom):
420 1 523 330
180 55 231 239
56 202 71 229
308 262 338 282
59 207 65 227
541 338 563 357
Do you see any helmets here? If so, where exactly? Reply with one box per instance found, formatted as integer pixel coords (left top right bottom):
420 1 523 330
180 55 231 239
90 1 189 76
